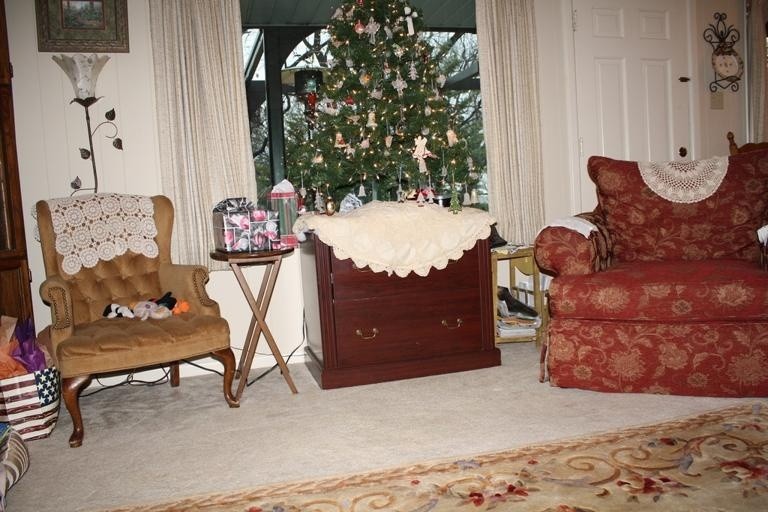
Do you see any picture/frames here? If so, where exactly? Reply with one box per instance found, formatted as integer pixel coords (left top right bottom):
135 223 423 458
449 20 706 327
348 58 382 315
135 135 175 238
34 0 130 54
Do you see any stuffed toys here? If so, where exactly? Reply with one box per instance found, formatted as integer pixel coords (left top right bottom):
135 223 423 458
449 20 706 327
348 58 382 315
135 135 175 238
102 292 190 321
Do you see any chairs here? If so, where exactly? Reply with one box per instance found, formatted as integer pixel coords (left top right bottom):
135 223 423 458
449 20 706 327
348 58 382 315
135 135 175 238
34 192 241 449
727 130 768 153
530 149 768 397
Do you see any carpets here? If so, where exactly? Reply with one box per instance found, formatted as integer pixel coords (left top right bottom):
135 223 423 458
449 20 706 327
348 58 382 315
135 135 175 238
109 397 767 511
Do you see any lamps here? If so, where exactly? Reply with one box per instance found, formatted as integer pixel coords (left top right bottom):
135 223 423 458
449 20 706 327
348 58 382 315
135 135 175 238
46 50 125 199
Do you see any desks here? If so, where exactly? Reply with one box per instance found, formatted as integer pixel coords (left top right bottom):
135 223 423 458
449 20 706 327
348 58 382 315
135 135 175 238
210 247 304 400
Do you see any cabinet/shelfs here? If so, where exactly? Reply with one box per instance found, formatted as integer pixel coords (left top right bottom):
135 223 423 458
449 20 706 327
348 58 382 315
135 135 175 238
300 211 502 390
0 0 32 341
492 242 549 346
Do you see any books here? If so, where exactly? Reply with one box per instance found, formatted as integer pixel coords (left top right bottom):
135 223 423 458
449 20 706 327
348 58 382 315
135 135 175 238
497 285 542 338
